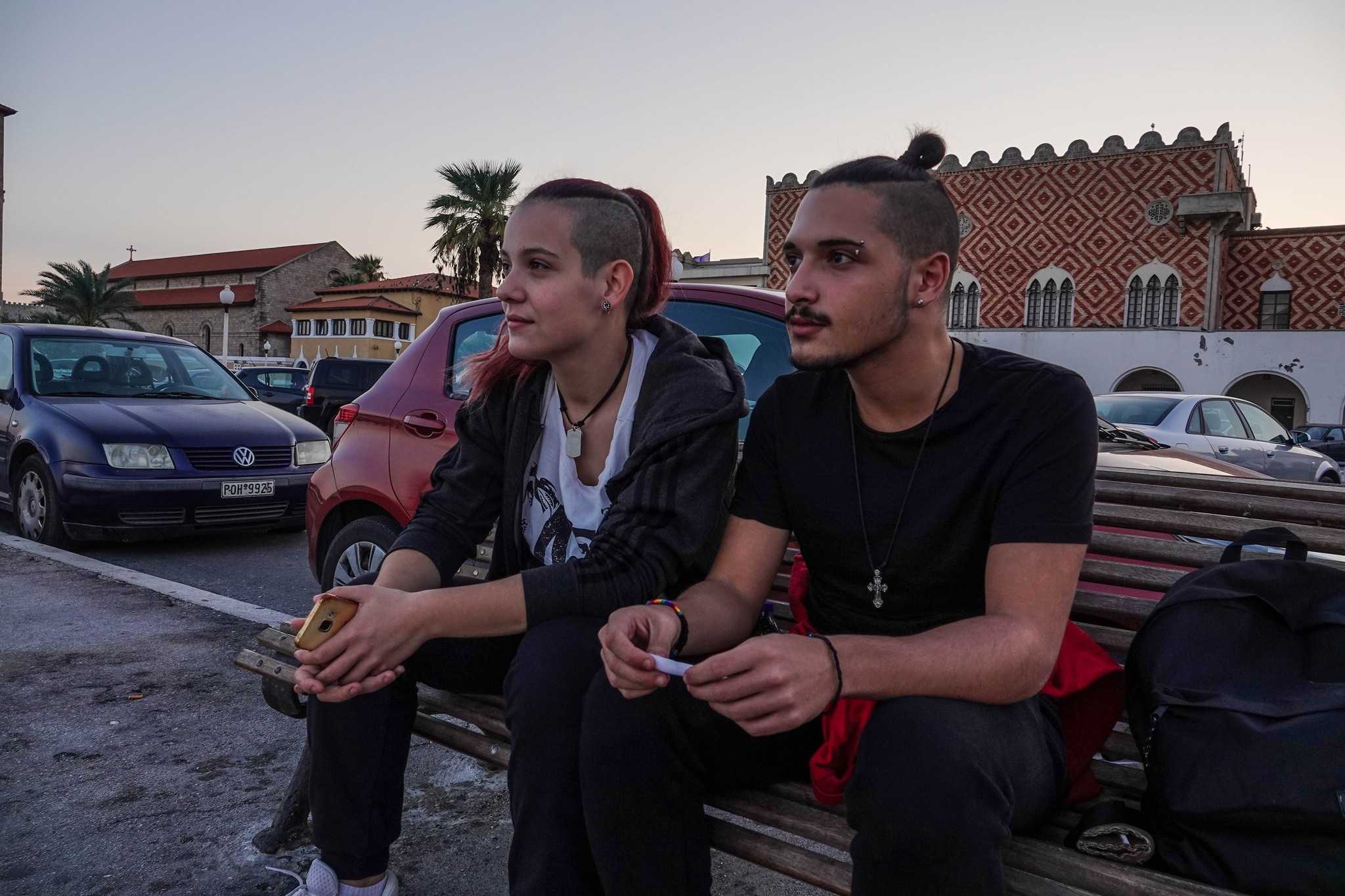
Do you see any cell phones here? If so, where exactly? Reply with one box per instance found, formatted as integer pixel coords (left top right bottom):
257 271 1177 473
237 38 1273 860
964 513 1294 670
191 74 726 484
294 593 359 651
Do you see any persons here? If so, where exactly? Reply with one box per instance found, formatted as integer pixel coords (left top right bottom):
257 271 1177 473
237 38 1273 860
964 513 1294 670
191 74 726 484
266 179 750 896
579 124 1099 896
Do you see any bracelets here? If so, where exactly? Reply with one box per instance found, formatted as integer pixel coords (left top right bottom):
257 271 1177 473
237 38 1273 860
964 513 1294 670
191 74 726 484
807 633 843 715
646 599 689 659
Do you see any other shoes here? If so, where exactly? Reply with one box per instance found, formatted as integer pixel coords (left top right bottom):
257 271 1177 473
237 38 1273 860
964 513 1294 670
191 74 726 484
264 859 398 896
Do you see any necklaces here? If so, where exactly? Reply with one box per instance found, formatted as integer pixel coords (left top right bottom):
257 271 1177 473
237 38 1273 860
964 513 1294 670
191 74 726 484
849 335 955 609
558 334 633 457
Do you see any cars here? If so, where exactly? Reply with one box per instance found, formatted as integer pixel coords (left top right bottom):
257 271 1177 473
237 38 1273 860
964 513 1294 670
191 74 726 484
34 350 398 449
1288 422 1345 465
1 321 334 548
302 277 1276 612
1093 390 1342 485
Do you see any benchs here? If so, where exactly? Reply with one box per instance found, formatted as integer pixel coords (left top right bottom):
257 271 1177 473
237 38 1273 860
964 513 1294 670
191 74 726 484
236 463 1345 896
32 353 119 394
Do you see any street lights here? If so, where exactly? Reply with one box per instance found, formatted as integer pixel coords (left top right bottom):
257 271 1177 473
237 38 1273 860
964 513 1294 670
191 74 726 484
219 283 235 370
264 340 271 382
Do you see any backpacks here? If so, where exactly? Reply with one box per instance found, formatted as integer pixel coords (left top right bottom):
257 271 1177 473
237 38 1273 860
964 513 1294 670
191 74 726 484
1124 525 1345 896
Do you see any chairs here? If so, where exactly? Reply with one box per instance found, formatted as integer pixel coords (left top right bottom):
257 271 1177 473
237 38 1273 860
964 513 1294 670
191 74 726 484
743 341 790 402
1191 410 1225 435
101 360 153 395
290 375 298 389
